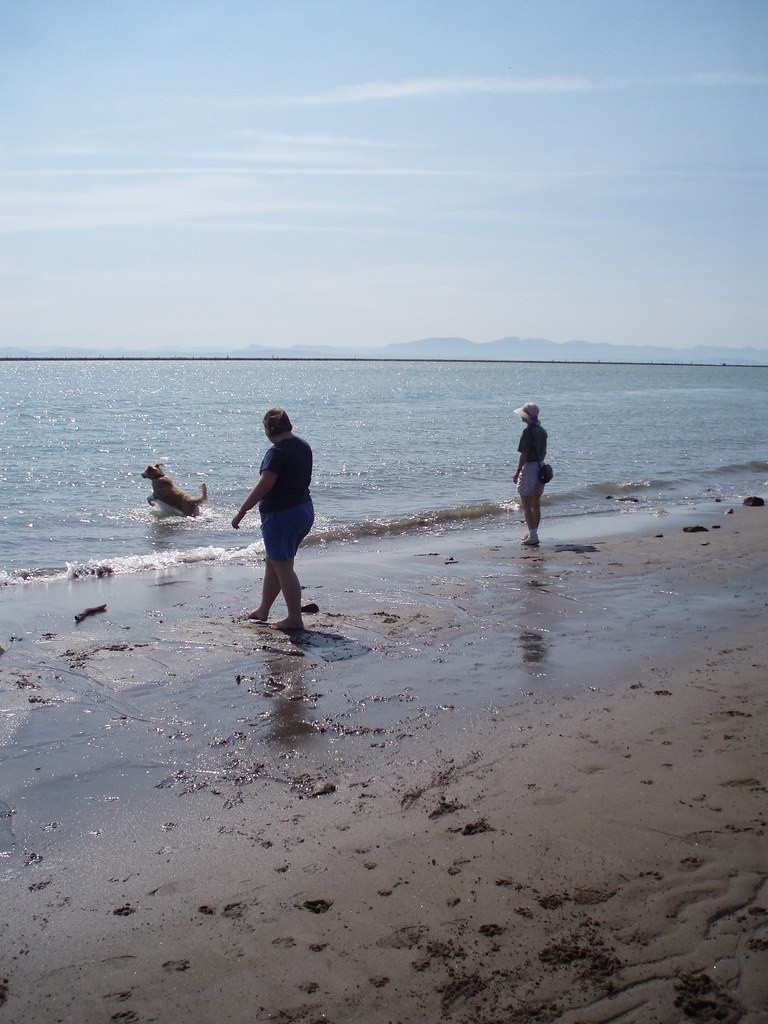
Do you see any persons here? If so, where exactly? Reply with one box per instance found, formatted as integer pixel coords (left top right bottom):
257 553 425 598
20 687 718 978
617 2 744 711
513 402 547 545
231 408 315 629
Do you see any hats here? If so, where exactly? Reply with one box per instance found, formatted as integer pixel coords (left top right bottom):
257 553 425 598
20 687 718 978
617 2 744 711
514 403 541 425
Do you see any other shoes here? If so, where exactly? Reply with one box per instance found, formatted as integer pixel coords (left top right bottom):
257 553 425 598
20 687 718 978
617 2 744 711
520 532 539 546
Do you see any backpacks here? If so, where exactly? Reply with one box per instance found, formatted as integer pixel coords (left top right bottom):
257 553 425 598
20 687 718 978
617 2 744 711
537 464 554 484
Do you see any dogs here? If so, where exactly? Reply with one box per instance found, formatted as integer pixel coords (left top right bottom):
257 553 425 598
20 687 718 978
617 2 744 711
142 463 208 517
